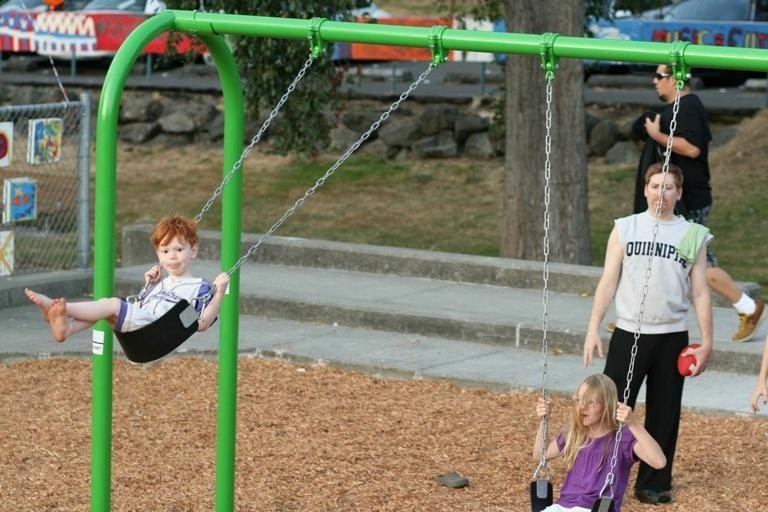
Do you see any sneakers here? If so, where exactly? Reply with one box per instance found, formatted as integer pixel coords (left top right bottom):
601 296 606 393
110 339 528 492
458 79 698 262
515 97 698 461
732 300 768 342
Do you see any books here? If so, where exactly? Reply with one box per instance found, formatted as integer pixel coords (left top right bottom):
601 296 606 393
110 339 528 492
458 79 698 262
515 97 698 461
0 120 14 167
0 230 17 276
26 118 62 165
3 176 37 222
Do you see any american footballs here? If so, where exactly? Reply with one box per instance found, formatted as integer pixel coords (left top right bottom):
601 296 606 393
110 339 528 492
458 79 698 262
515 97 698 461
677 344 702 376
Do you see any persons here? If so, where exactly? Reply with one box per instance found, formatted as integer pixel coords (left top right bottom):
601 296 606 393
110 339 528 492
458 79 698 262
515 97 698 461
751 328 768 412
583 165 715 505
534 373 668 512
24 215 229 347
629 54 765 344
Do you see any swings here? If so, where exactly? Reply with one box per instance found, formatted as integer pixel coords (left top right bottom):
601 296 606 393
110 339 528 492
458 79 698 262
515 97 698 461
114 39 433 362
531 67 685 512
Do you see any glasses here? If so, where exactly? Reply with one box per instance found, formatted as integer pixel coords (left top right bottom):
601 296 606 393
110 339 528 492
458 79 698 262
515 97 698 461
656 70 671 81
